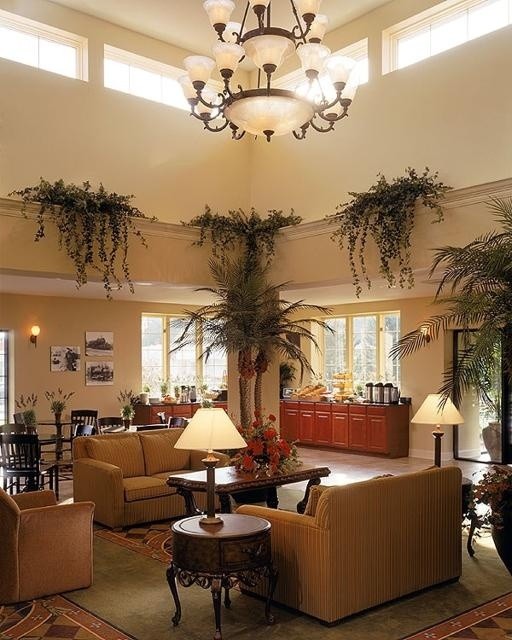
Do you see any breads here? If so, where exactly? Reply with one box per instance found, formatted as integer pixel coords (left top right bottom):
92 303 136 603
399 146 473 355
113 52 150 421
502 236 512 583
291 385 326 400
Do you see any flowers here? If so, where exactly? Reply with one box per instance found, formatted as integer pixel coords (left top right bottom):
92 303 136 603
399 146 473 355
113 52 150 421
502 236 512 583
235 416 302 475
463 466 512 533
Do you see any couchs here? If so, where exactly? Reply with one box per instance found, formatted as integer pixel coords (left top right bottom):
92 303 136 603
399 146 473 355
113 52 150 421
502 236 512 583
237 464 463 628
70 428 234 531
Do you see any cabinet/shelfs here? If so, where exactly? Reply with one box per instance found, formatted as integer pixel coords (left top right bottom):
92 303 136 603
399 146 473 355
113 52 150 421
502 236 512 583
281 400 410 459
133 399 228 425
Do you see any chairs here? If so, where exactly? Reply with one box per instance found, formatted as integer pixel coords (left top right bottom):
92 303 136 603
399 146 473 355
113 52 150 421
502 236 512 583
0 491 95 604
0 410 124 501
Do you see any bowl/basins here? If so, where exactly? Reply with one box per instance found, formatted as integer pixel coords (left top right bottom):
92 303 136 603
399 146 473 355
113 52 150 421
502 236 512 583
150 398 161 404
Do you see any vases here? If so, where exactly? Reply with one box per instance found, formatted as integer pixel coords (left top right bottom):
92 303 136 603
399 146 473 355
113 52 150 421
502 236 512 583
123 419 130 428
491 526 512 574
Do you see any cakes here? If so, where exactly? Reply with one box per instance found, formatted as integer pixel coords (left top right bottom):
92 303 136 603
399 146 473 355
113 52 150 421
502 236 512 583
333 373 353 400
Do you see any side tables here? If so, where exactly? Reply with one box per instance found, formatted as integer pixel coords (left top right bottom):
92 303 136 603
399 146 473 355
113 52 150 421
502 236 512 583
462 476 476 557
164 513 277 640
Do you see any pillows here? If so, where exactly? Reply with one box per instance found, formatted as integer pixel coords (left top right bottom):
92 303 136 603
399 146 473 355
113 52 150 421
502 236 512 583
305 485 335 517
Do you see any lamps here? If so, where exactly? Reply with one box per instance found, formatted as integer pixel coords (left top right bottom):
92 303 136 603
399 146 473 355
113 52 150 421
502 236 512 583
412 393 464 467
30 324 40 343
183 0 360 141
174 408 248 524
419 327 430 343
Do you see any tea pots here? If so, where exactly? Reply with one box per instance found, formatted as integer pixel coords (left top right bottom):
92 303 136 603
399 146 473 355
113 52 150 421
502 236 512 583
180 385 189 403
390 386 400 403
192 386 198 403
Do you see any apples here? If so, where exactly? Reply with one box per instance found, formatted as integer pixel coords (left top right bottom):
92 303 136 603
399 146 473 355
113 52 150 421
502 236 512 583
165 397 175 401
